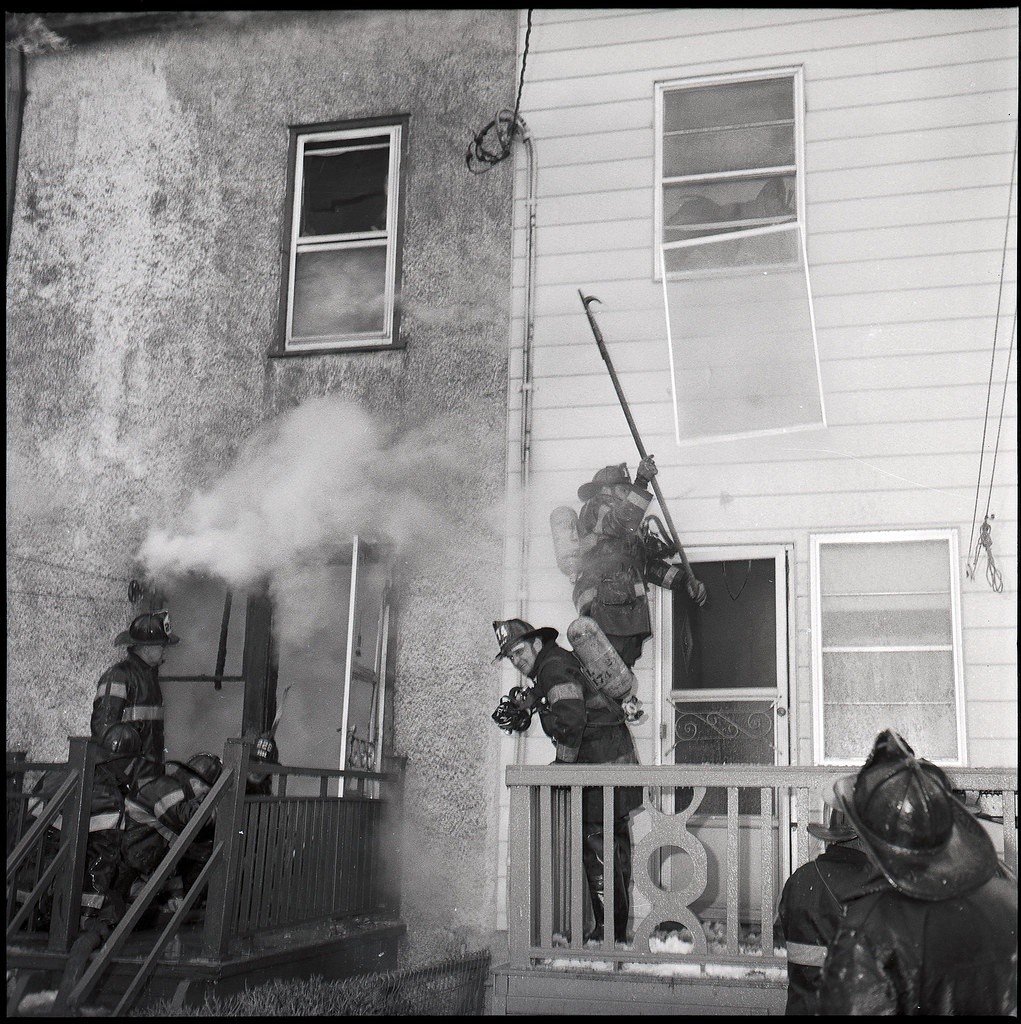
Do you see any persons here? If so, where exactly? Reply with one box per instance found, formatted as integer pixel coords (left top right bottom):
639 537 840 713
489 619 643 945
777 728 1019 1018
39 613 282 934
570 453 707 667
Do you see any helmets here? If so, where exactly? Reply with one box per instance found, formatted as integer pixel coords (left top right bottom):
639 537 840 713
832 729 999 902
93 720 147 765
808 805 858 840
578 465 634 502
113 614 181 647
165 752 222 786
491 620 560 663
229 734 279 764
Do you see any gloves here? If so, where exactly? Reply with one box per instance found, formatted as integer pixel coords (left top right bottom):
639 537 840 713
637 454 659 485
686 579 708 608
